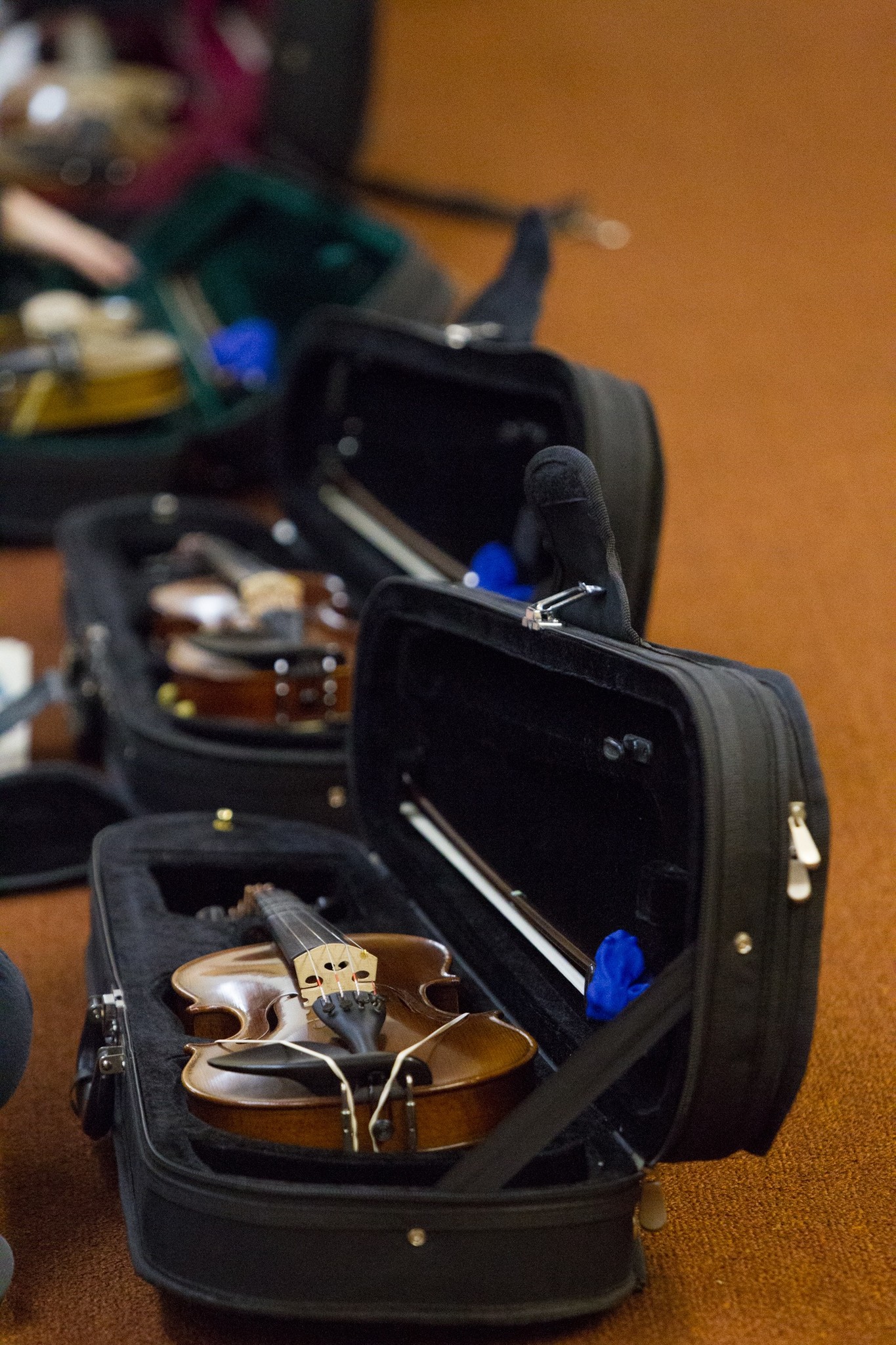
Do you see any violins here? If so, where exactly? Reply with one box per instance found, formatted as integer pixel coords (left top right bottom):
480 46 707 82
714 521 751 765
143 527 360 732
168 882 538 1159
